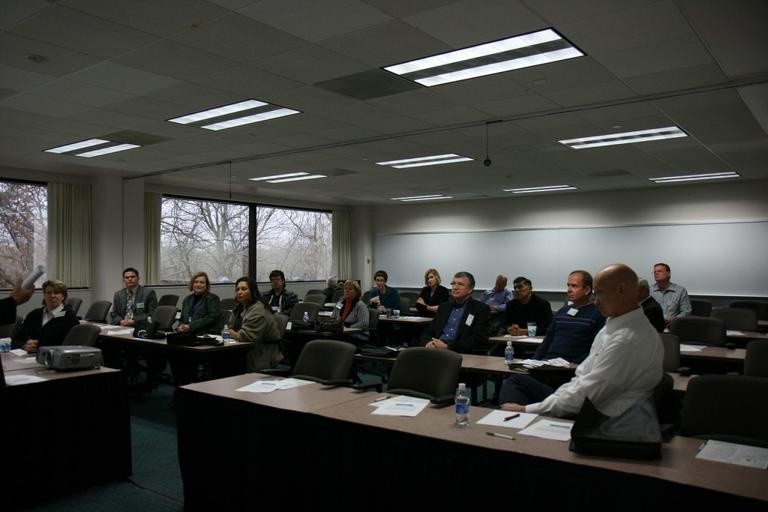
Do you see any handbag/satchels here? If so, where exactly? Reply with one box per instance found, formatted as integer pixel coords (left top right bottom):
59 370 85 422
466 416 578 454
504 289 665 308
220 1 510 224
167 331 218 347
569 395 662 460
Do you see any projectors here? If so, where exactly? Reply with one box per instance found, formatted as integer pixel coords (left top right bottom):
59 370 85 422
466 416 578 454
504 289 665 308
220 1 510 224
37 344 101 371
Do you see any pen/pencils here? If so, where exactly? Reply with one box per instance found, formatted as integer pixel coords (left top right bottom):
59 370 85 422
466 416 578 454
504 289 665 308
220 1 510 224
485 431 515 440
505 414 520 419
697 442 705 452
375 396 391 402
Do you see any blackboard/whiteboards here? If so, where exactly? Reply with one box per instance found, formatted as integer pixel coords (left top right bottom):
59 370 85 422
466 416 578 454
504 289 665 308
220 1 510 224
372 218 768 299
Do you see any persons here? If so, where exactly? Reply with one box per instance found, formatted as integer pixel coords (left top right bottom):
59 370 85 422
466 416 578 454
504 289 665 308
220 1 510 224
262 270 299 315
110 268 157 326
177 273 224 335
227 277 285 373
361 270 400 347
500 263 663 419
404 269 449 347
649 263 691 328
0 265 44 396
502 270 605 407
12 280 80 355
330 280 370 353
637 277 667 334
420 271 492 389
478 274 553 359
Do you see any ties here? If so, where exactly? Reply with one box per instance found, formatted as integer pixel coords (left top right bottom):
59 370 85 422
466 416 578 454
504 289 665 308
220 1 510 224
124 292 134 319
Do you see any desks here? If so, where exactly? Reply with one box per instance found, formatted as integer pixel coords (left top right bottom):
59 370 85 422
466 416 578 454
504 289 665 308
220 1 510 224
175 373 767 511
0 349 131 511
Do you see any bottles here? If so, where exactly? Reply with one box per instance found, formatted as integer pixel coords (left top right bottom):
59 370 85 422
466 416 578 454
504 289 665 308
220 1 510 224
222 324 229 344
504 341 514 364
386 308 391 318
455 383 471 425
303 311 309 322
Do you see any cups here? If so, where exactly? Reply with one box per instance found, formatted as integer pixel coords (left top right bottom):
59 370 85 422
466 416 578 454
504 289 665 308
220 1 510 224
527 322 537 338
393 310 400 318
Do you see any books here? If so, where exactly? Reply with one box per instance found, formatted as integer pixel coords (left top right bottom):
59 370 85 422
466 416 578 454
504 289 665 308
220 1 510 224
21 264 46 290
